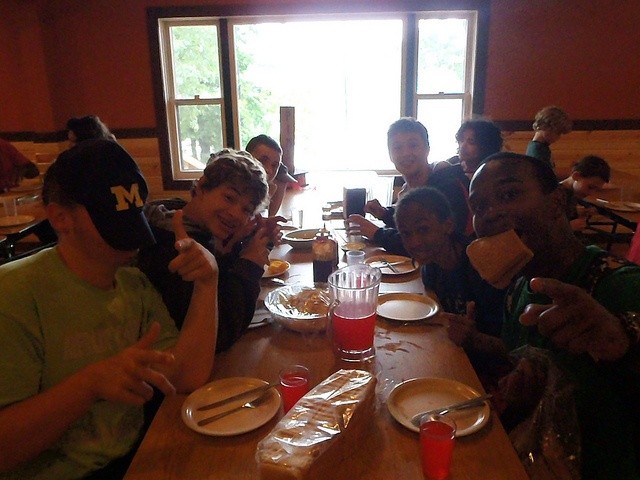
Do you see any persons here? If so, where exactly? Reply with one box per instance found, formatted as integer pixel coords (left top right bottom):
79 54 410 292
526 106 572 193
345 119 474 258
394 187 482 347
192 148 258 254
131 154 287 425
1 139 220 479
0 138 39 189
445 120 502 178
245 135 287 217
66 114 117 149
467 152 640 479
560 156 610 232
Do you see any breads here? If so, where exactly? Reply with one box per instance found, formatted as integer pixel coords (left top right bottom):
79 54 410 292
466 229 534 291
258 369 377 479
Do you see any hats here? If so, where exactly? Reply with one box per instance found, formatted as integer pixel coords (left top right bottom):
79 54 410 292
274 163 298 184
57 138 158 251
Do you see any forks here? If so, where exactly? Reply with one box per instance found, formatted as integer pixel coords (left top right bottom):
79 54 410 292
381 257 400 273
197 390 274 427
250 318 274 325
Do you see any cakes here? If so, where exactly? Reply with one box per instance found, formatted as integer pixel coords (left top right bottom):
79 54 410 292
268 260 290 274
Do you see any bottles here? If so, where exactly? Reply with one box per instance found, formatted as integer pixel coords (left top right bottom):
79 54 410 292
312 233 338 286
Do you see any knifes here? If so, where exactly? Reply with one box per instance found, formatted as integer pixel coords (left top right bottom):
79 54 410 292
196 380 280 412
378 258 412 268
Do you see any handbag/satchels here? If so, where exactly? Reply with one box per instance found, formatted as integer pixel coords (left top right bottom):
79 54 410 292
489 343 583 479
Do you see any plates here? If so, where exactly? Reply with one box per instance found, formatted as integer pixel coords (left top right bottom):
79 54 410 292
260 258 291 278
0 215 36 227
604 201 640 212
363 254 420 276
183 378 280 436
385 376 491 437
342 242 365 251
376 292 440 321
247 300 274 329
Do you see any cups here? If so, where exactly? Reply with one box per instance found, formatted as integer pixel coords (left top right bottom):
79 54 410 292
621 184 632 201
419 413 457 480
278 365 310 415
326 263 383 363
348 222 362 243
291 209 303 229
3 197 18 216
346 250 366 266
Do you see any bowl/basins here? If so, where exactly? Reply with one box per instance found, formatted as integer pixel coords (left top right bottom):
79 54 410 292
281 229 318 249
264 285 330 334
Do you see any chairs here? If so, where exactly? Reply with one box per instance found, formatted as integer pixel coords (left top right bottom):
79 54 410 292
1 241 57 266
152 200 186 210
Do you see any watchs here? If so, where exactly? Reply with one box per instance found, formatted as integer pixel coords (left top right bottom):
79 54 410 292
615 309 640 348
